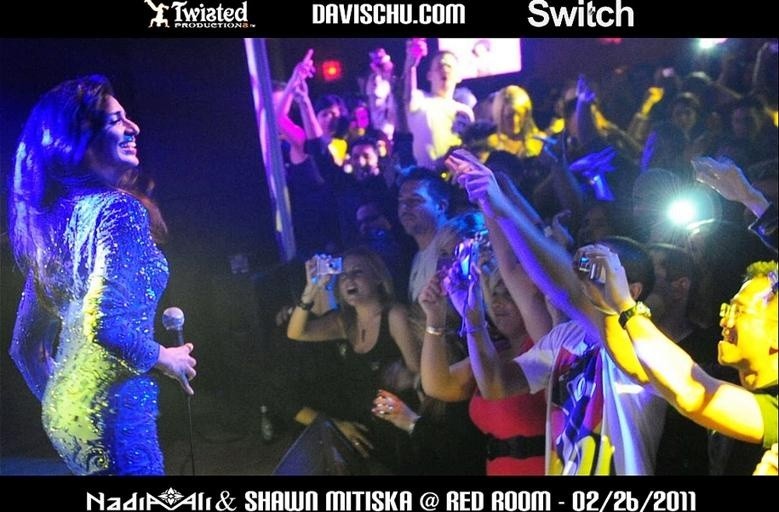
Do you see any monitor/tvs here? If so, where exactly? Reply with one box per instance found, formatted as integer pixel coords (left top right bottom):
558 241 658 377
432 37 527 87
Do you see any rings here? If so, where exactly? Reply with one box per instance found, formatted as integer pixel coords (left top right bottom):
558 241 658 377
176 373 188 383
185 342 194 350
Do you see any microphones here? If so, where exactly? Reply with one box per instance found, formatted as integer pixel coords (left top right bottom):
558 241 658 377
162 306 192 398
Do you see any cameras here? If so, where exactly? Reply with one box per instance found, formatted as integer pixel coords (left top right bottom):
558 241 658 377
315 257 344 277
452 239 478 279
435 267 462 295
575 250 603 282
472 230 494 253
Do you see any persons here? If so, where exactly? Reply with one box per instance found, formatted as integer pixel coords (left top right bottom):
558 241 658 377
6 73 200 478
261 39 778 479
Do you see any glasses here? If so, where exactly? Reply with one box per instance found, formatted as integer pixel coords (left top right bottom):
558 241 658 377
719 303 748 319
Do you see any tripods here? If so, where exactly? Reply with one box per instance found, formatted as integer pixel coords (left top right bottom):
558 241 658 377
269 410 365 477
